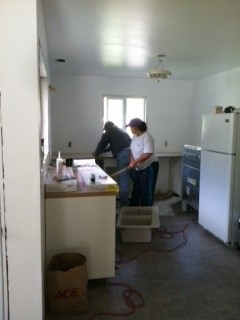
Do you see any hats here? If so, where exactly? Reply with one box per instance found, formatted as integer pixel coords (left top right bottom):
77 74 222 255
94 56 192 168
125 118 142 127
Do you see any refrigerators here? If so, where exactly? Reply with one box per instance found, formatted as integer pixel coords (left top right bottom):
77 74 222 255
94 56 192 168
198 113 240 248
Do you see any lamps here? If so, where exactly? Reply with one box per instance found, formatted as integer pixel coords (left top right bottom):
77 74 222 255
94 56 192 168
146 58 172 83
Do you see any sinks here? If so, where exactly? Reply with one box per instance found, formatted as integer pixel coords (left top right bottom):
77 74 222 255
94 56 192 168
116 206 160 243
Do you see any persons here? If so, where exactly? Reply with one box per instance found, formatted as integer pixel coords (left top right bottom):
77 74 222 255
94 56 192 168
93 121 132 208
125 118 159 207
